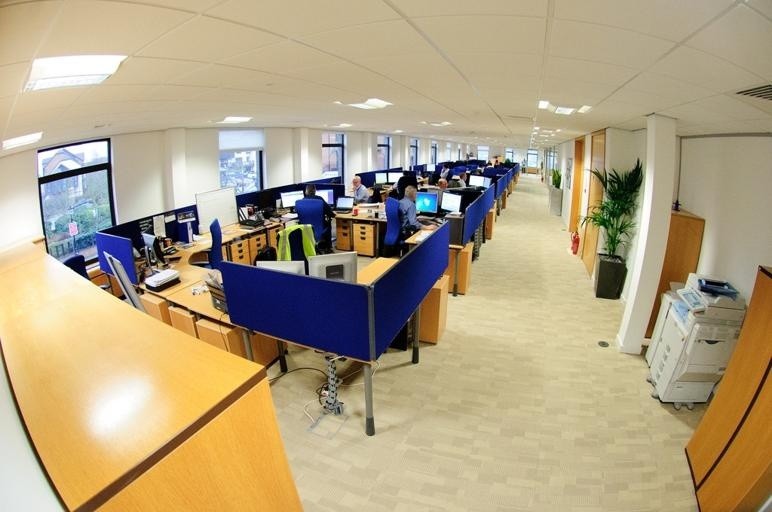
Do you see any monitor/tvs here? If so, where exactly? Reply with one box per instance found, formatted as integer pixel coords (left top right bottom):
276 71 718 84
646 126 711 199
469 175 484 186
441 191 462 212
415 192 438 214
141 233 166 277
426 164 435 171
280 190 305 213
388 172 404 183
315 189 334 205
256 260 305 274
308 251 358 283
375 173 387 184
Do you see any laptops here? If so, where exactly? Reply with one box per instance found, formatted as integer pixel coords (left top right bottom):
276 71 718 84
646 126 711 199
333 197 355 211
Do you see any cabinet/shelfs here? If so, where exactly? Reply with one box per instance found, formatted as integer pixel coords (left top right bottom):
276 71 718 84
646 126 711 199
684 265 772 511
644 213 705 338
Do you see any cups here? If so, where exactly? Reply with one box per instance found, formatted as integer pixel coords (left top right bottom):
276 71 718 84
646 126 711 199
375 210 378 218
165 238 172 246
417 185 420 190
368 209 372 217
354 208 358 215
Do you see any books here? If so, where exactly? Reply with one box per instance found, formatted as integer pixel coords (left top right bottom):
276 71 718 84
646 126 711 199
240 219 263 230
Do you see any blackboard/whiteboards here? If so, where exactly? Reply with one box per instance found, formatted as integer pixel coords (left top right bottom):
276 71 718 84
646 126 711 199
194 185 241 235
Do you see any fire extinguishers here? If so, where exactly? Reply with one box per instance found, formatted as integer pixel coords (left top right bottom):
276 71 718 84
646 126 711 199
570 233 580 255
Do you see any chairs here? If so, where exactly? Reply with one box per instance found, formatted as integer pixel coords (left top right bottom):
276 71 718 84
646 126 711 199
63 255 110 289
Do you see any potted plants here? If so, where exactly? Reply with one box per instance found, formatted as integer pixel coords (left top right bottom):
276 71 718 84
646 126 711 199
581 158 643 299
549 170 562 215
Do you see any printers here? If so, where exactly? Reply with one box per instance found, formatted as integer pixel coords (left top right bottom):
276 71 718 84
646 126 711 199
676 272 746 321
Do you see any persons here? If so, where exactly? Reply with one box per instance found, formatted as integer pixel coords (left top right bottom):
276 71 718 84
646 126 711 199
388 169 409 195
476 156 500 173
303 184 333 254
439 163 450 178
398 184 436 234
350 175 371 203
457 172 467 187
434 178 450 206
